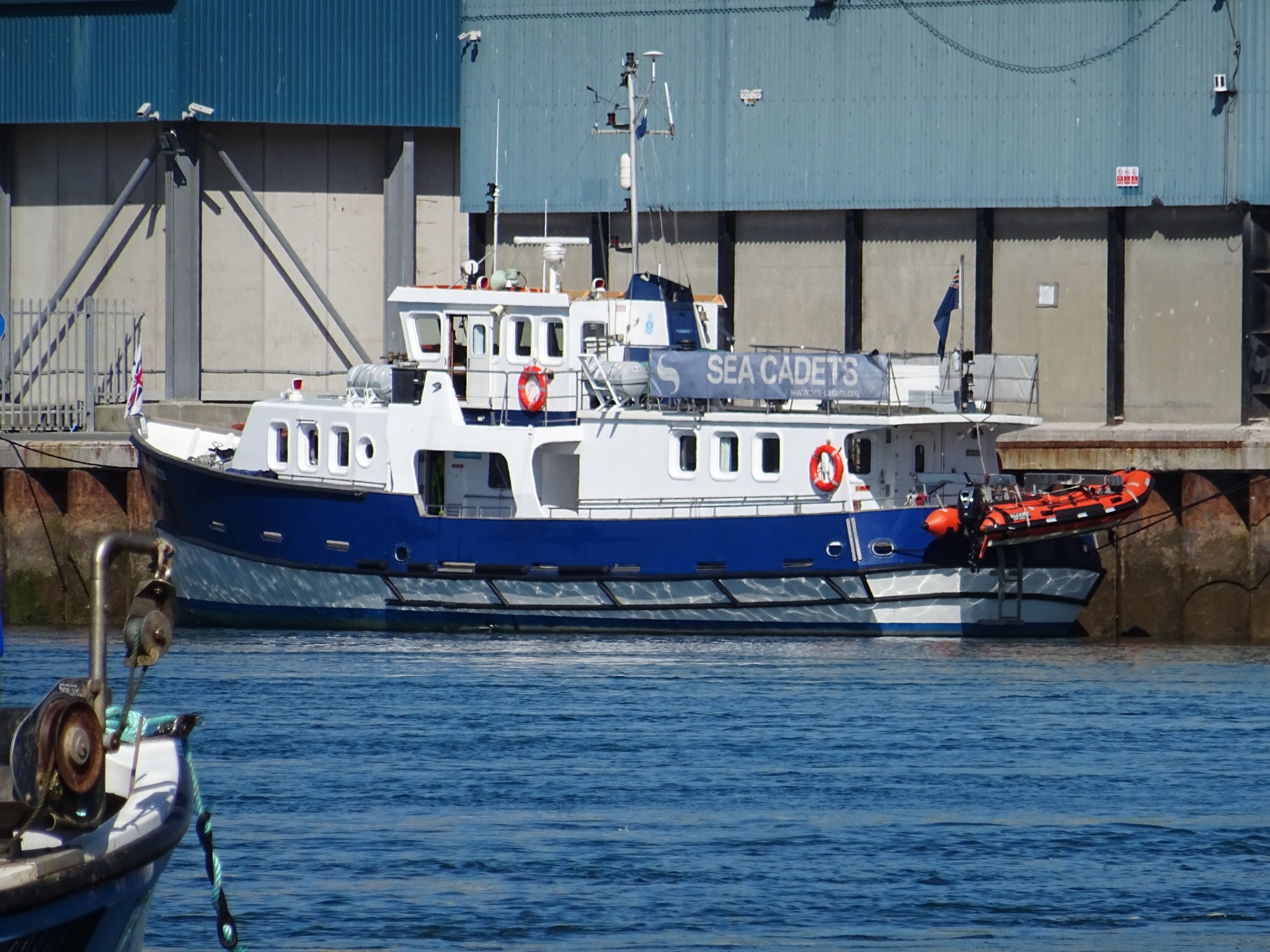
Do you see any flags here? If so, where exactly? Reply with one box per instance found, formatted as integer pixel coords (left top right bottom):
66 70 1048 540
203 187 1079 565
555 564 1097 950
933 263 962 362
124 339 143 420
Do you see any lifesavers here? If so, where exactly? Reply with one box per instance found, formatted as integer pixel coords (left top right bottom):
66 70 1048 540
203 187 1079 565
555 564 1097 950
810 445 844 493
518 365 548 413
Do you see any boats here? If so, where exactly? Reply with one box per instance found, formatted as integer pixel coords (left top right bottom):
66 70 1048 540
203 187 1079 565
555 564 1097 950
921 466 1155 552
0 535 248 951
123 52 1161 633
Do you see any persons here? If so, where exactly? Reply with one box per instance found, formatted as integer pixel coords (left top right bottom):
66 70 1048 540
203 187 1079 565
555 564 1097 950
956 482 987 567
